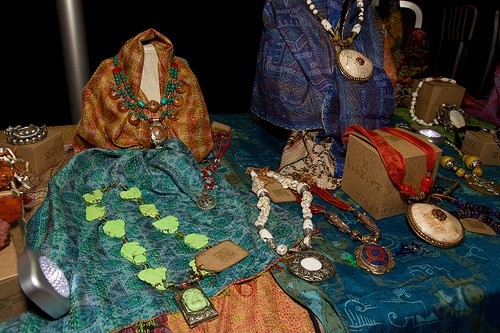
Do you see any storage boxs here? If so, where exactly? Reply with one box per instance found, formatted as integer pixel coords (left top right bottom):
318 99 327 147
461 130 500 166
409 77 466 121
339 125 443 220
0 130 64 175
0 226 35 322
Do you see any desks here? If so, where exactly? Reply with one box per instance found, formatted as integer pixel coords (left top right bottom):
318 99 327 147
0 106 500 333
